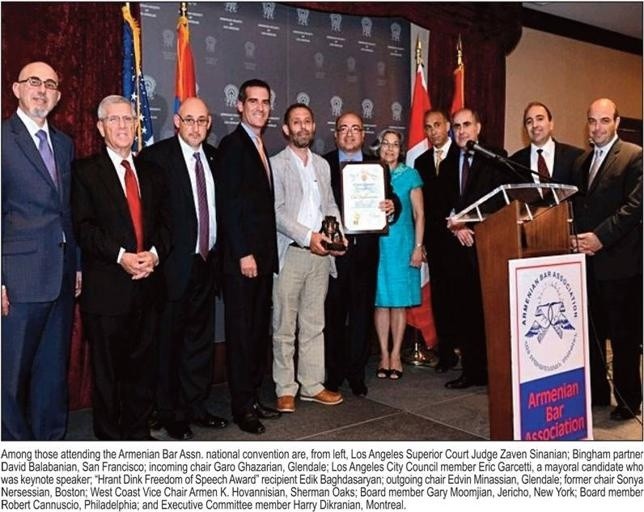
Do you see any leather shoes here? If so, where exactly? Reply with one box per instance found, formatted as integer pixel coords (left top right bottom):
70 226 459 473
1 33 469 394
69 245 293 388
445 370 486 389
610 403 641 420
434 352 459 374
253 400 281 420
298 388 343 406
162 418 194 441
348 372 368 399
232 406 265 434
190 411 228 430
275 395 297 414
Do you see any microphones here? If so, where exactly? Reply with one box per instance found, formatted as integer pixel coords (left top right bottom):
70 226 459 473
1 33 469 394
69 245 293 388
467 140 496 157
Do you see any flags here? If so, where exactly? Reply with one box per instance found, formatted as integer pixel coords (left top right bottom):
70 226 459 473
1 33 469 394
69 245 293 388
172 18 197 134
121 5 156 152
406 65 445 351
449 63 465 117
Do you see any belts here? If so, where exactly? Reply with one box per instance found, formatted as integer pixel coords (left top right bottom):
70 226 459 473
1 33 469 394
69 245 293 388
290 242 311 251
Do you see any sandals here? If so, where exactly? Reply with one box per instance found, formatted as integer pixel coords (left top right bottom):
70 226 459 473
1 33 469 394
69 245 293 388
376 362 390 380
388 367 403 380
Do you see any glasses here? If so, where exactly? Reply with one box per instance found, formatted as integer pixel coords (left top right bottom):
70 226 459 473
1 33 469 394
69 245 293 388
380 140 401 147
336 124 363 135
175 111 209 126
19 78 63 90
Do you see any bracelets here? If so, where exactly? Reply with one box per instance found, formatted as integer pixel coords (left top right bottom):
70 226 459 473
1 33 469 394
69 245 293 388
415 243 422 247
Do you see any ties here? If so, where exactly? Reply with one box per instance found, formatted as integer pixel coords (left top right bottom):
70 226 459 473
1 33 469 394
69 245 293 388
588 150 603 188
121 160 144 253
536 150 552 203
256 136 267 173
192 151 210 260
461 151 473 199
434 148 442 173
35 130 58 194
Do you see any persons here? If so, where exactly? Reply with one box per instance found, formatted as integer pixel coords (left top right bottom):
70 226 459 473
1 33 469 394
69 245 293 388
414 109 458 373
1 61 83 440
568 98 642 419
71 94 171 441
438 108 509 389
134 96 230 440
322 112 402 399
508 101 586 204
219 79 281 433
267 103 346 412
373 128 427 380
